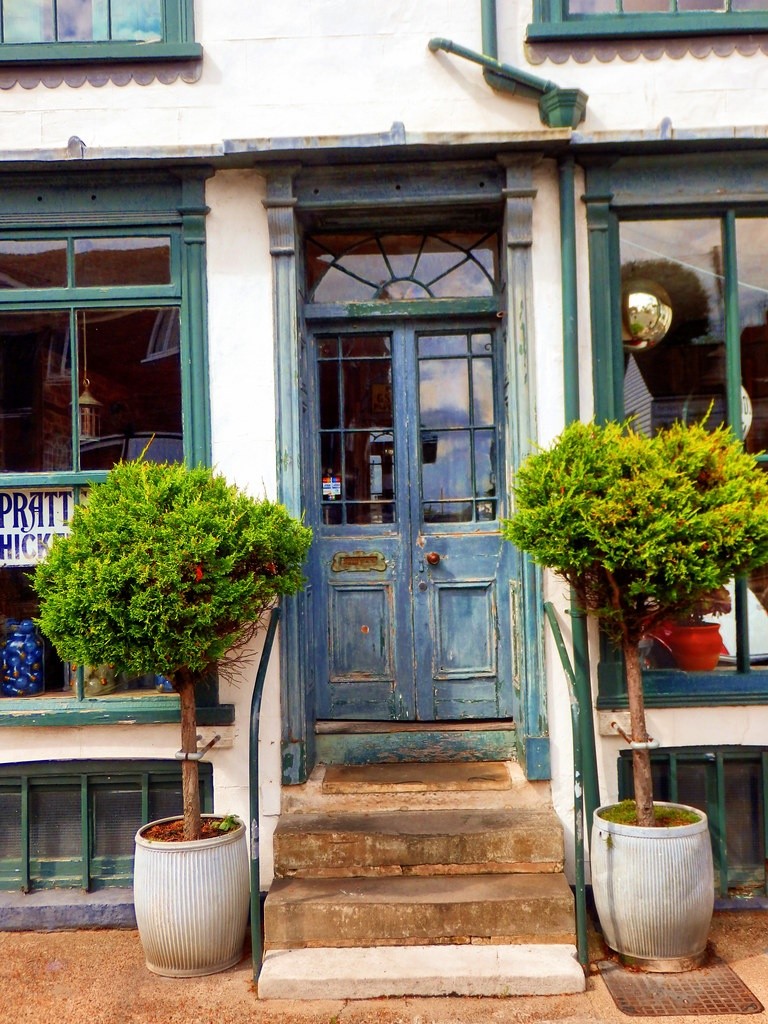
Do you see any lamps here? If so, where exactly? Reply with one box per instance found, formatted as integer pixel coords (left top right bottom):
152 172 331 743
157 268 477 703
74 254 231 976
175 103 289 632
69 311 103 442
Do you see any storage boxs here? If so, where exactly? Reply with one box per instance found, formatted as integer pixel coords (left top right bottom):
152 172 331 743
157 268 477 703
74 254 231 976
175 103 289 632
322 483 341 495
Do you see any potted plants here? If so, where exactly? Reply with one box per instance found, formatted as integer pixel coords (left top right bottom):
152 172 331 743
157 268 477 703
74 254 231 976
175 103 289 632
669 585 732 671
23 464 311 979
501 424 768 975
